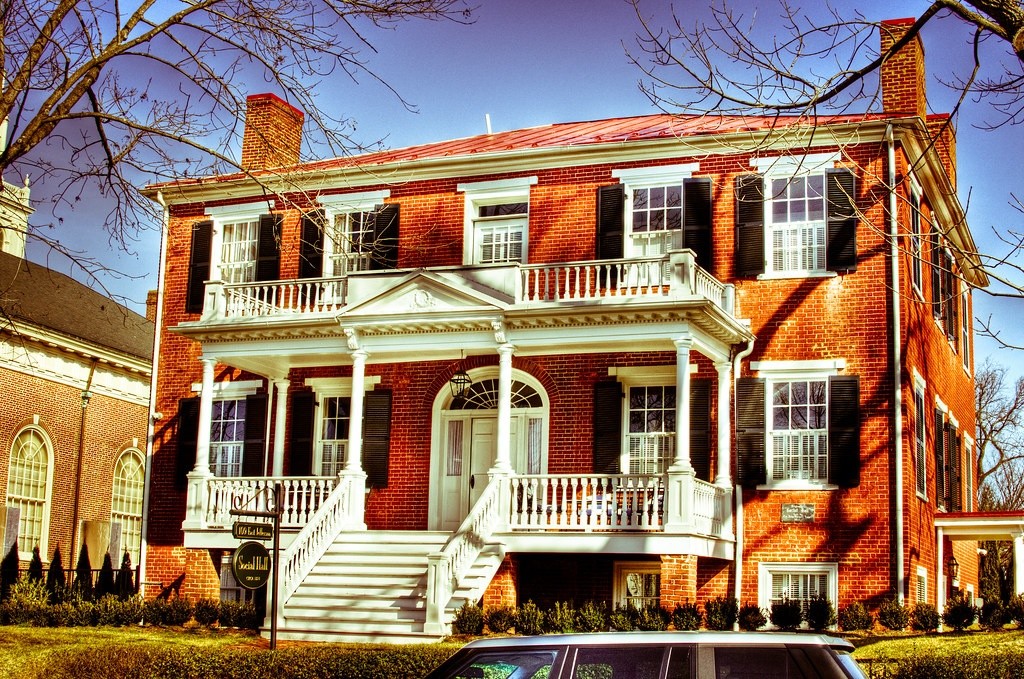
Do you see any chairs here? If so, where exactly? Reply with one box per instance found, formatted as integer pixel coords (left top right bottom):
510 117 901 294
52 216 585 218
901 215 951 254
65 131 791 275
515 480 663 528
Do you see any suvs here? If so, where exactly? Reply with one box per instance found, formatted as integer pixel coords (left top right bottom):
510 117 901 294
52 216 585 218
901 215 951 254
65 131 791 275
424 631 868 679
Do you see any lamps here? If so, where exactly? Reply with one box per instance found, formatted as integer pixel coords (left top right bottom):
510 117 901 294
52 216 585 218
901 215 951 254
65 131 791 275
450 349 473 399
